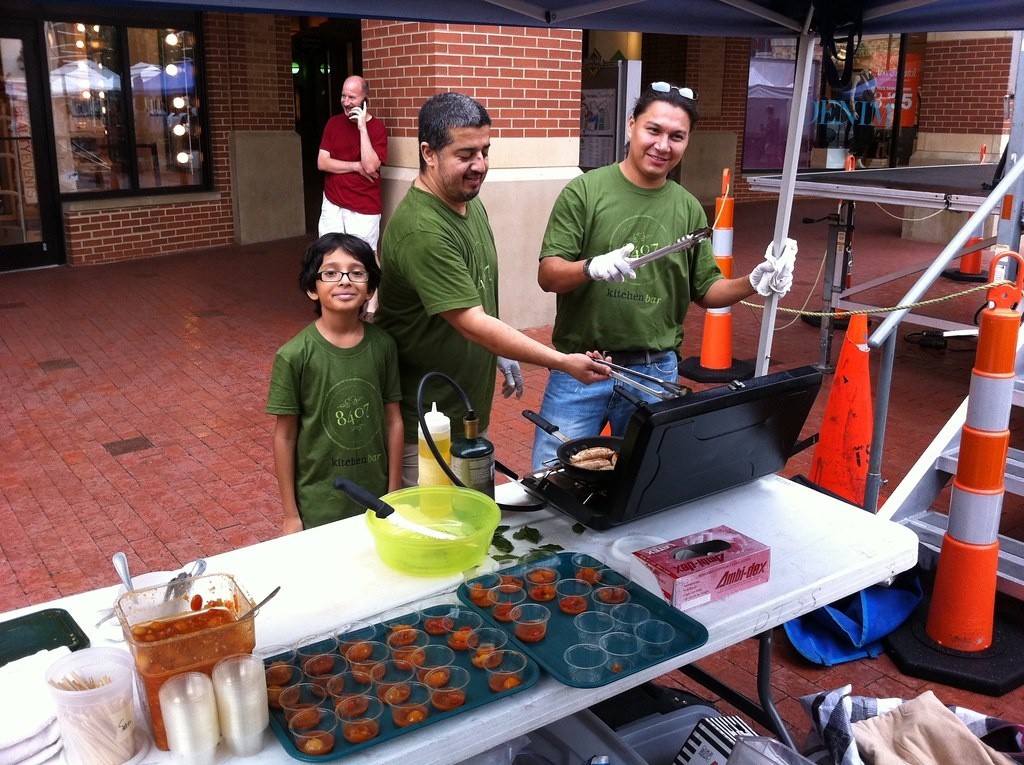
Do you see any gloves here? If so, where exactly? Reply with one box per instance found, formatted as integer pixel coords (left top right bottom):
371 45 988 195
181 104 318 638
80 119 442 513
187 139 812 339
589 243 645 283
497 354 523 400
749 255 794 291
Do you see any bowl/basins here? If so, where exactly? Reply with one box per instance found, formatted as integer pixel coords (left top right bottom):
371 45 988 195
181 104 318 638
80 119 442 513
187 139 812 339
365 484 501 578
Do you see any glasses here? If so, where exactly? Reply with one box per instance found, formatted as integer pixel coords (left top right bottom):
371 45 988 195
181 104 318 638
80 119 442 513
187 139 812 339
646 81 699 100
316 271 371 283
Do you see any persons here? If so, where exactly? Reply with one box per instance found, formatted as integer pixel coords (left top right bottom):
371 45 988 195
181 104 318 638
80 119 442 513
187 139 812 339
318 75 388 323
844 55 878 169
375 92 614 488
266 232 404 535
532 81 775 471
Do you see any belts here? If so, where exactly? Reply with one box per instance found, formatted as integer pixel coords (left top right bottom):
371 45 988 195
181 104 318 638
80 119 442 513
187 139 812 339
607 351 670 366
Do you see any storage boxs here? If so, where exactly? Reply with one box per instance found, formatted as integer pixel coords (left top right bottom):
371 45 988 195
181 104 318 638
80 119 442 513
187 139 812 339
510 704 725 764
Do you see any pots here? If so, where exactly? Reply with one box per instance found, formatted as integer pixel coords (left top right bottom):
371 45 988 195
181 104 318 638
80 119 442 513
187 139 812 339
522 409 624 481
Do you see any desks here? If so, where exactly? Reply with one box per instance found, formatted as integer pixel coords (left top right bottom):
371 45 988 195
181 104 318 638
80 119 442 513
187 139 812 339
0 473 917 765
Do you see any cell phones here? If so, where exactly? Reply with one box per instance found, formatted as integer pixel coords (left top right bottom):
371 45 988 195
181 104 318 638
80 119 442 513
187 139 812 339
349 97 369 117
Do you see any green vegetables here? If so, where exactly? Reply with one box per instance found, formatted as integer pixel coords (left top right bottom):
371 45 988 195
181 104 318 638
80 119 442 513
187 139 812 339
488 523 585 565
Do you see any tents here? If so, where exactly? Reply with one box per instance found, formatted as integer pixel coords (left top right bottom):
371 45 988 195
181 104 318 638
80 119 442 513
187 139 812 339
148 0 1024 380
49 58 194 95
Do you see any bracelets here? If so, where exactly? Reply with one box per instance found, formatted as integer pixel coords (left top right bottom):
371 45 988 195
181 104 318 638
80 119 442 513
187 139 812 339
583 257 593 279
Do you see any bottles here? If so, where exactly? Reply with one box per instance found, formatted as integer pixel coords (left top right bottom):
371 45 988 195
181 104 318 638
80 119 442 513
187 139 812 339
417 402 451 487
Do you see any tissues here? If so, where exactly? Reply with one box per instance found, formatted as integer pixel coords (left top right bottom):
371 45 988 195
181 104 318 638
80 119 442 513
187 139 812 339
629 525 771 612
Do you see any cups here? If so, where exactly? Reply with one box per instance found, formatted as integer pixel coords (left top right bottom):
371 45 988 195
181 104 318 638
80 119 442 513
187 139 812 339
465 550 675 683
159 653 269 765
255 595 526 755
42 647 135 764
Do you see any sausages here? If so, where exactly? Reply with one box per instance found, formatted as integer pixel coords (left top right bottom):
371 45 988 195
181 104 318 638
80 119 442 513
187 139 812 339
570 446 618 470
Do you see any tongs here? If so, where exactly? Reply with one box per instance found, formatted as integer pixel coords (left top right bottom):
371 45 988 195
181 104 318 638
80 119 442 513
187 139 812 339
629 226 713 269
590 358 693 401
